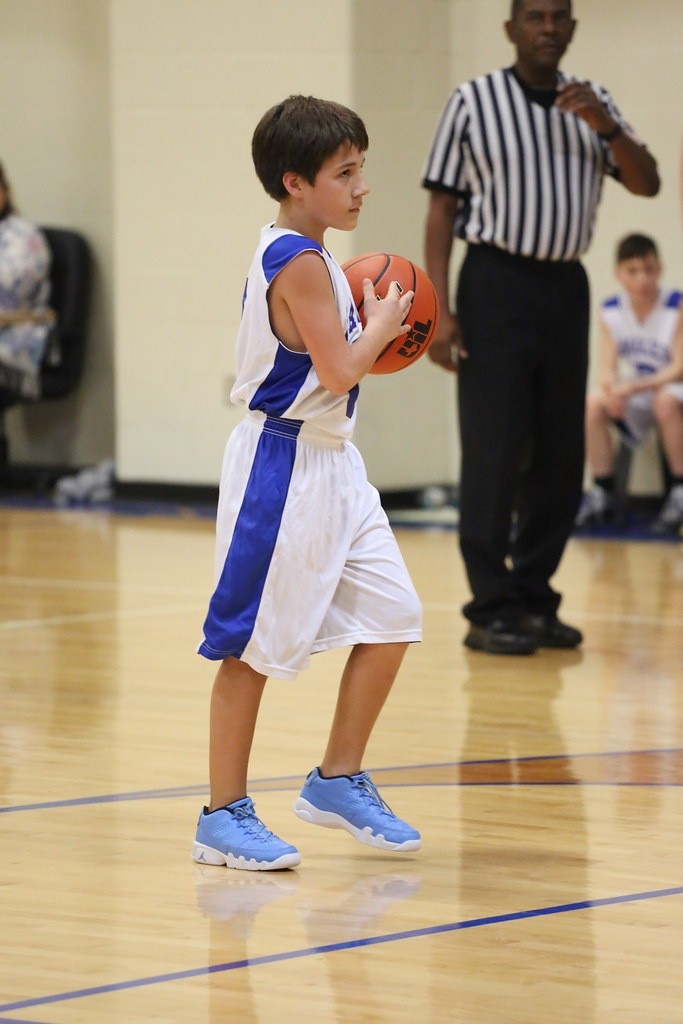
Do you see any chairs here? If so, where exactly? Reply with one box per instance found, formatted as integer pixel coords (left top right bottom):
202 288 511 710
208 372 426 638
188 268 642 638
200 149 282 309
1 227 98 412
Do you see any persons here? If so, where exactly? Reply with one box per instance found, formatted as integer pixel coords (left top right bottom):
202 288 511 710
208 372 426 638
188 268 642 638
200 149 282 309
188 89 420 869
429 2 659 654
576 231 683 529
0 160 59 407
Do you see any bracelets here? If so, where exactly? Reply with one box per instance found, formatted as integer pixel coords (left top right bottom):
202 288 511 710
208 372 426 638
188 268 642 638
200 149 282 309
599 124 622 144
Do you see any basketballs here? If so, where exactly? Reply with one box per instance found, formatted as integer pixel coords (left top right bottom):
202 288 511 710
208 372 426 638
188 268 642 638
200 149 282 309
340 253 440 375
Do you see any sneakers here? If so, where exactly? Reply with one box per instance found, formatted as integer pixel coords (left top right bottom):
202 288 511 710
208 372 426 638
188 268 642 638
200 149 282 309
293 768 422 852
188 798 302 872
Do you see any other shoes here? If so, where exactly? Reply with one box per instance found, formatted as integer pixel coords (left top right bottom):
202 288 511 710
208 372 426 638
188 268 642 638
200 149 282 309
580 486 682 531
466 616 581 653
